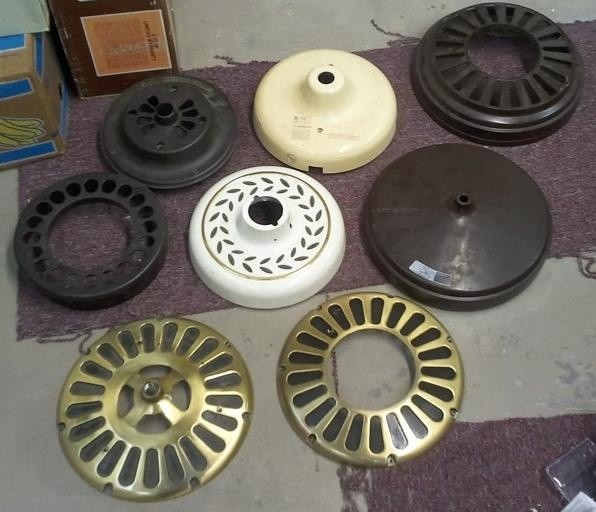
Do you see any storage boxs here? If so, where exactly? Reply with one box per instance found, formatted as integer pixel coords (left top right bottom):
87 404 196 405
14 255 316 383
0 31 70 171
0 1 52 36
46 1 178 101
